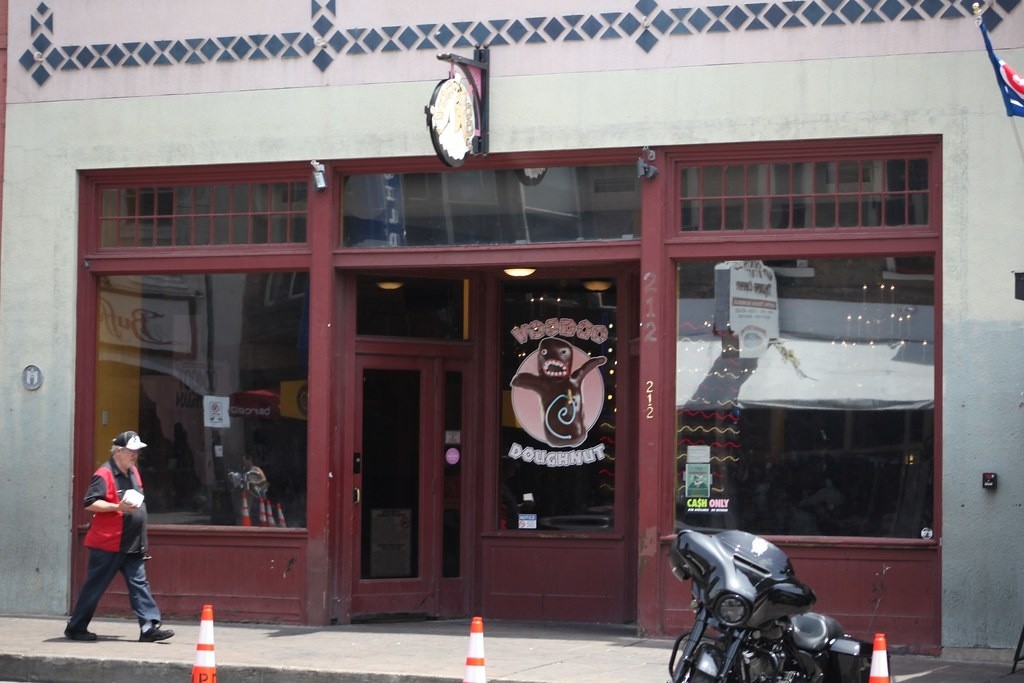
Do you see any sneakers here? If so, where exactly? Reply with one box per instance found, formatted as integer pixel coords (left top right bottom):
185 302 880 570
65 625 97 641
139 627 174 642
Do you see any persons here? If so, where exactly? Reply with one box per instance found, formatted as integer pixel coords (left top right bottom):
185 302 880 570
227 455 266 526
64 430 175 644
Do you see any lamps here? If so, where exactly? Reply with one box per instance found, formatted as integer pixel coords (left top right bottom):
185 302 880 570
310 160 328 192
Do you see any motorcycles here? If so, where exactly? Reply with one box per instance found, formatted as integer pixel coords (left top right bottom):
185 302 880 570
665 528 872 683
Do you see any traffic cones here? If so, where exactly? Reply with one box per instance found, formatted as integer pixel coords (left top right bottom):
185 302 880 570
461 615 486 683
866 633 891 683
258 498 269 527
191 604 216 683
277 502 287 528
240 492 253 526
266 499 276 527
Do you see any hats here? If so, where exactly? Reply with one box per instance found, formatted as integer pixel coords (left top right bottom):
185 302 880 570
113 430 147 450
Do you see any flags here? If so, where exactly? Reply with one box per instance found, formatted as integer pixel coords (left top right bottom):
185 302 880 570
978 17 1024 116
